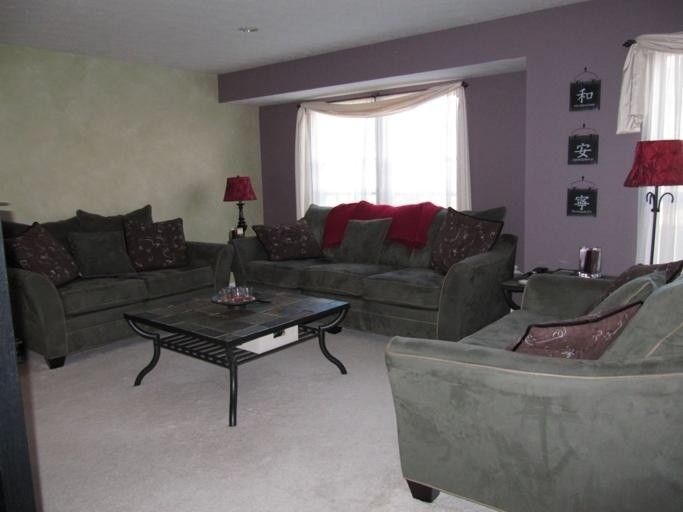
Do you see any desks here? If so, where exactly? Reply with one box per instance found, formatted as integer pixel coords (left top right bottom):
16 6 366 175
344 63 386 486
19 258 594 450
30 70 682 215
498 267 618 312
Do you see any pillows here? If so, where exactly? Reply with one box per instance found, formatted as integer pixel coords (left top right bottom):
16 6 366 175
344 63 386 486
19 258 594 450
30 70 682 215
3 221 80 288
251 202 506 274
61 228 136 276
121 216 187 273
76 204 152 226
579 257 682 317
506 299 642 361
1 217 76 242
588 268 669 317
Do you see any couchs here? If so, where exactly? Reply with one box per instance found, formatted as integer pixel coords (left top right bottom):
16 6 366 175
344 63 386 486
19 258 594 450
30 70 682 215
227 203 520 343
1 203 234 370
382 268 683 511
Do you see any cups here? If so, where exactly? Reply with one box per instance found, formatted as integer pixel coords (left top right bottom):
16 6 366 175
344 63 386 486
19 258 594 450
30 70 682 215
219 285 252 302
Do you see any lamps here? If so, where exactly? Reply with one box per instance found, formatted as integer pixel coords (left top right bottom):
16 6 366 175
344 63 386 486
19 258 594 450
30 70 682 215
622 138 683 264
221 174 258 234
